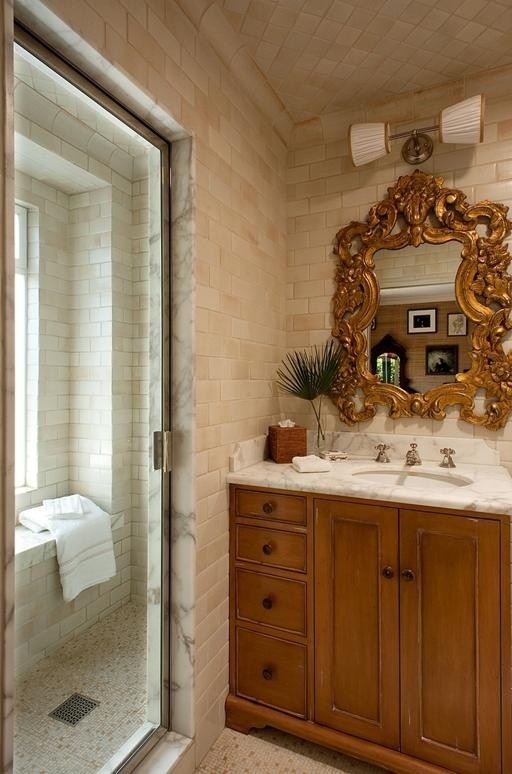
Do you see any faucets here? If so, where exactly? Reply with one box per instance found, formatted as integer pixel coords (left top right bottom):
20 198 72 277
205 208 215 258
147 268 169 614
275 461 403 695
407 444 422 465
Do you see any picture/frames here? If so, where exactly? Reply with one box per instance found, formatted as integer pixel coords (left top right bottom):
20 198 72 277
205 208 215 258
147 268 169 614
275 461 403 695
426 344 458 375
447 313 467 336
407 309 438 333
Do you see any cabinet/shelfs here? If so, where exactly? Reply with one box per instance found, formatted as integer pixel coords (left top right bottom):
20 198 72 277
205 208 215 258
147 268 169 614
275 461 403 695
224 482 313 736
314 493 511 773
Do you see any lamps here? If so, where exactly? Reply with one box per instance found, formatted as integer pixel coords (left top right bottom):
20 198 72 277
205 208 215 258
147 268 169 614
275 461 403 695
348 92 485 165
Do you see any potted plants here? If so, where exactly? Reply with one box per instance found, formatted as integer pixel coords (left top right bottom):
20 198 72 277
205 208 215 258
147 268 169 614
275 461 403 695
275 339 356 457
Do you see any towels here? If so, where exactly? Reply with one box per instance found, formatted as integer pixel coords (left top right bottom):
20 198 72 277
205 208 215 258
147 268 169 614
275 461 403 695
18 493 118 602
292 455 330 472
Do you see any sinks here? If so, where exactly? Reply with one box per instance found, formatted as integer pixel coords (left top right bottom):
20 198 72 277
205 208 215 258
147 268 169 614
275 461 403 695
350 465 473 490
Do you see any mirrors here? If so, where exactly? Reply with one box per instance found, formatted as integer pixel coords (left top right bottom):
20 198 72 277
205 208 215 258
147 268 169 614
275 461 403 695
371 333 415 393
326 168 511 432
